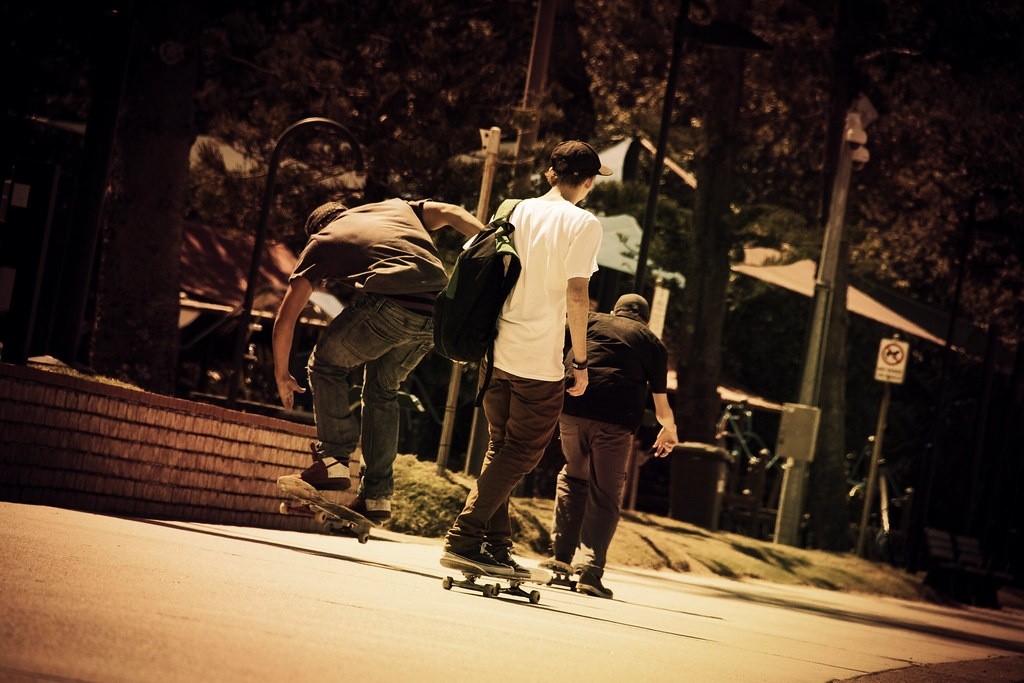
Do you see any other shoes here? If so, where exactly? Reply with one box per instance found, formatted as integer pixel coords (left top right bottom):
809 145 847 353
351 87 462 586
554 549 573 565
575 565 613 599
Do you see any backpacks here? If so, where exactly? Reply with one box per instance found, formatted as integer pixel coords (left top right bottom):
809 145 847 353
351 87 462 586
432 199 522 365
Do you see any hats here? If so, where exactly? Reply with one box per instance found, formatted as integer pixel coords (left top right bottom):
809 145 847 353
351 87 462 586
614 294 650 324
550 139 614 176
305 202 348 236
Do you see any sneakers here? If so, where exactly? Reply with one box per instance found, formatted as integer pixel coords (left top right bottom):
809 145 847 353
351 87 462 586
439 535 515 576
341 475 391 520
484 535 532 578
276 442 351 504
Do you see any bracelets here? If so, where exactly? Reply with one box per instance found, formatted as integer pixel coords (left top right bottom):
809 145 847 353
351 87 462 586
572 358 589 370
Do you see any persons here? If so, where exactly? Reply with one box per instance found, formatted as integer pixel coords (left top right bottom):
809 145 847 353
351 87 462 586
886 487 914 567
565 294 601 329
442 141 614 577
550 293 679 600
271 197 487 523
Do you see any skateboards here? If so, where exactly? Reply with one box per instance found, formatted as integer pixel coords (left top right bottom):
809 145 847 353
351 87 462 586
440 553 548 604
274 473 383 543
537 561 579 593
